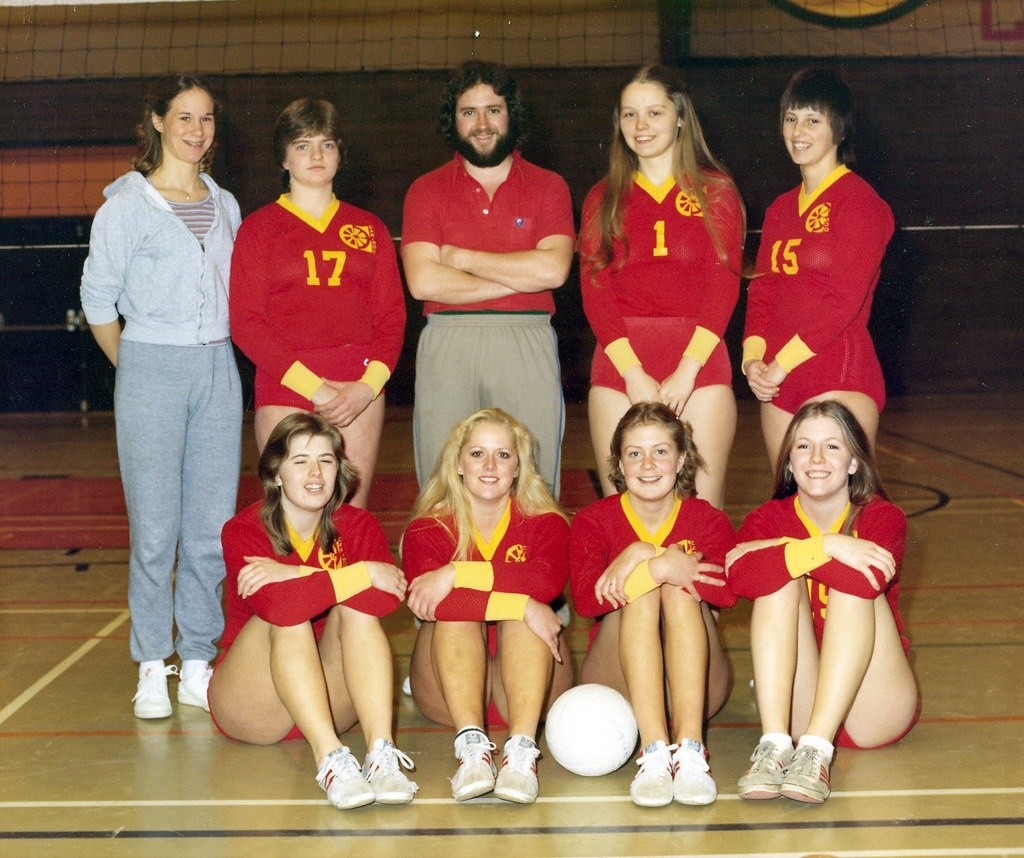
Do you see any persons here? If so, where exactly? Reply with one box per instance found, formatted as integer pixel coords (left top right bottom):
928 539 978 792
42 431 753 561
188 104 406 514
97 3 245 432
401 410 578 809
206 411 420 810
401 62 577 507
740 69 893 486
725 404 921 804
228 98 405 516
81 78 250 721
578 66 740 525
572 402 735 809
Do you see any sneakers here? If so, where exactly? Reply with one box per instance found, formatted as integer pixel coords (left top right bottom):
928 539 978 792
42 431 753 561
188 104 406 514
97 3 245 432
674 743 721 805
362 747 418 804
629 743 674 807
782 748 834 802
133 663 174 720
176 667 217 712
316 747 374 811
737 740 796 801
450 739 498 798
495 739 543 805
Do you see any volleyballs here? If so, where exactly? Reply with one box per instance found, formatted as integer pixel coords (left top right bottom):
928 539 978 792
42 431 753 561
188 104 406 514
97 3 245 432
546 683 638 776
